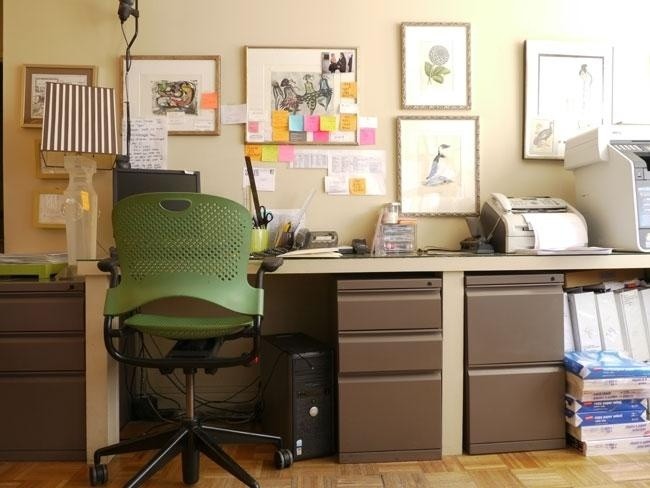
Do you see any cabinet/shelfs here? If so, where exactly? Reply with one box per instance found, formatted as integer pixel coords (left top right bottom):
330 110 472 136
462 274 568 456
1 279 87 462
329 277 443 465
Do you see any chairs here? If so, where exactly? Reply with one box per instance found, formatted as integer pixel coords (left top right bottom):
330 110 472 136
88 192 294 488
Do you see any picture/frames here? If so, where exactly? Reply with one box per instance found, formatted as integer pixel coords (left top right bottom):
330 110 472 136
32 187 67 231
245 44 360 147
524 39 615 160
19 64 98 129
35 140 80 181
396 114 480 217
120 54 222 136
398 21 471 111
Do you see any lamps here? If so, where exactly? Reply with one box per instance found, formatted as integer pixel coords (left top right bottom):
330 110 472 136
41 83 119 265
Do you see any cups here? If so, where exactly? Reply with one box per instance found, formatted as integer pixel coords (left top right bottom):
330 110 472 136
250 229 269 252
282 231 294 251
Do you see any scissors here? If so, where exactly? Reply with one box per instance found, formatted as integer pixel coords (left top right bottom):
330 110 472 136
258 206 273 229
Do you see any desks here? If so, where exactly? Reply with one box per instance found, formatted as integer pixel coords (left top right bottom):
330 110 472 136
72 251 650 456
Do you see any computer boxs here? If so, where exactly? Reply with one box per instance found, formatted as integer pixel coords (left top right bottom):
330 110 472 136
258 332 335 462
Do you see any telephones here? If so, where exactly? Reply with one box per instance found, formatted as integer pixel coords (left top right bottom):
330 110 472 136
296 229 338 249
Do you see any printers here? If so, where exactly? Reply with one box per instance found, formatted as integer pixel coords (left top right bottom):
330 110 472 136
466 193 589 255
564 126 650 254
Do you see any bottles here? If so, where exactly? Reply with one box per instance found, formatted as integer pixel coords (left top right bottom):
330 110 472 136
382 202 400 225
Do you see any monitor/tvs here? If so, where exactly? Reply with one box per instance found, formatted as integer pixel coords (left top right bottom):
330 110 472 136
113 167 200 241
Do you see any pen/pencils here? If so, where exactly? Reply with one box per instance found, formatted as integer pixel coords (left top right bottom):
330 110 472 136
285 222 291 233
252 217 258 229
